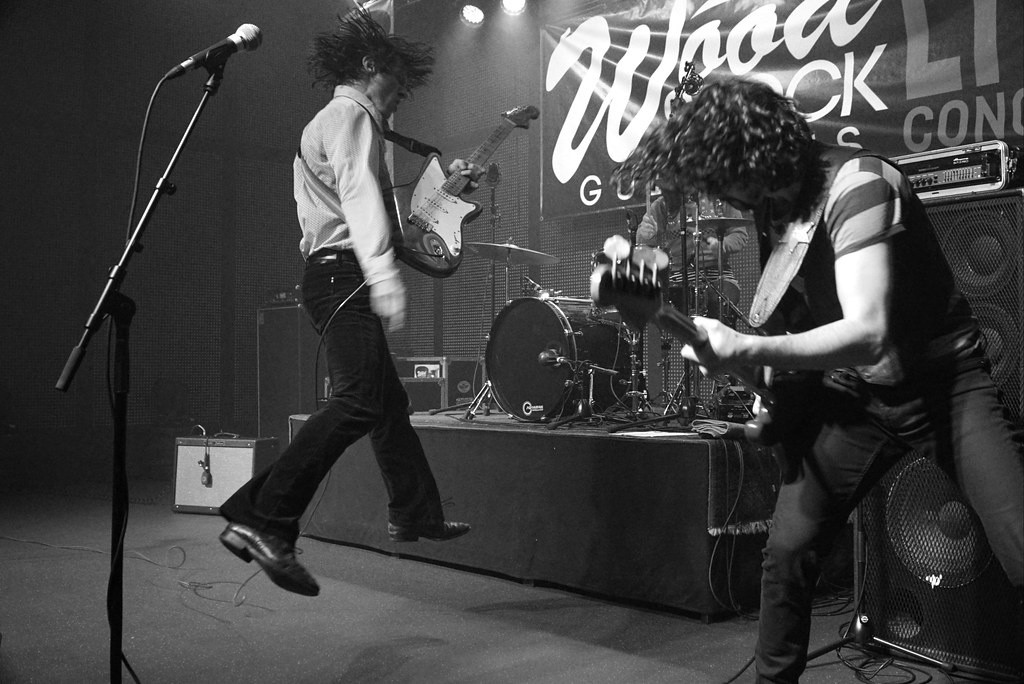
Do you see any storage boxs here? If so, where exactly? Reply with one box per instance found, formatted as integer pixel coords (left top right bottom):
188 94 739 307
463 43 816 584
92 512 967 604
325 356 485 413
170 435 281 515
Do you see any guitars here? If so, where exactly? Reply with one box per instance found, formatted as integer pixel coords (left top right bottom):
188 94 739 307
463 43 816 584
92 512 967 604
378 103 542 280
588 232 825 475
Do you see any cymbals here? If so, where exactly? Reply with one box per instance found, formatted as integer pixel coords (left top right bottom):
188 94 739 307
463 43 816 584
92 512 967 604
464 241 560 267
679 214 755 231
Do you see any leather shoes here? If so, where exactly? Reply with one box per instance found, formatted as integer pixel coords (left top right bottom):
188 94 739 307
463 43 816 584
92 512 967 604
219 520 319 596
387 520 472 543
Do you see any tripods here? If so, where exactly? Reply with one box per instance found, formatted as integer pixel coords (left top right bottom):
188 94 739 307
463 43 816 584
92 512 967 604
606 208 724 434
543 370 651 431
806 505 959 674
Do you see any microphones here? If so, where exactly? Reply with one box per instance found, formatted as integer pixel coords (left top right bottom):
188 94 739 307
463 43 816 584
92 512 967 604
163 21 265 80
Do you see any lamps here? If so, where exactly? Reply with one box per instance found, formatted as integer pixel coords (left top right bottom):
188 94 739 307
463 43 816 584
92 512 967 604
458 0 528 28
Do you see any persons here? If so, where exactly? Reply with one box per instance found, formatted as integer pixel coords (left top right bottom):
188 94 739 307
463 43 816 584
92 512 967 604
611 79 1024 684
218 7 485 597
635 188 754 387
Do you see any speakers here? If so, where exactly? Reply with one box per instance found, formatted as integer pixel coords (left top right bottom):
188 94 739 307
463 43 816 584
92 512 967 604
170 436 280 515
396 355 486 412
853 189 1024 684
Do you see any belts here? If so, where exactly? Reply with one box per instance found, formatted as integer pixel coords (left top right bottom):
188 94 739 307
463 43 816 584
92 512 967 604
306 250 355 268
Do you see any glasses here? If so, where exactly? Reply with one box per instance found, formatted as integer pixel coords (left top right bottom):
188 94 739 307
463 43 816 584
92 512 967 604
377 67 407 87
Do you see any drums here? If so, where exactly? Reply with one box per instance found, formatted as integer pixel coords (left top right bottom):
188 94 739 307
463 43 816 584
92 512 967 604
484 294 643 423
664 280 708 318
591 242 653 269
